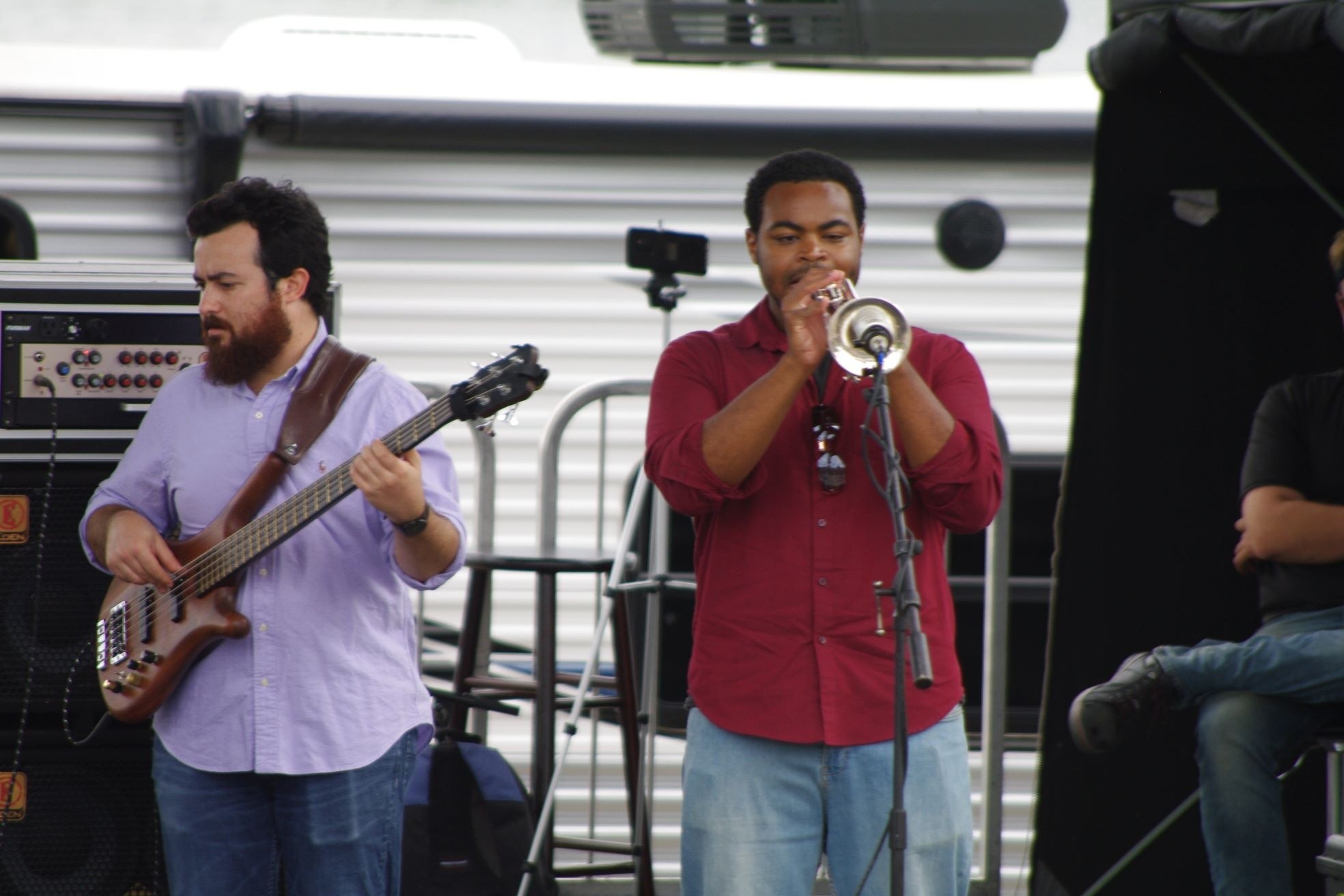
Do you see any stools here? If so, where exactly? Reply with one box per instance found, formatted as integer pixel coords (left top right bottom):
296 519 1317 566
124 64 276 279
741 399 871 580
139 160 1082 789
419 548 638 896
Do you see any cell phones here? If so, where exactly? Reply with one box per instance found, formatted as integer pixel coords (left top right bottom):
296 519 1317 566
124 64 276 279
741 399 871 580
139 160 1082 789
626 227 709 277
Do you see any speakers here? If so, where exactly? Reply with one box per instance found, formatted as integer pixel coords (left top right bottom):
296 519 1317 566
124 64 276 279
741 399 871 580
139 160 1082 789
0 484 175 896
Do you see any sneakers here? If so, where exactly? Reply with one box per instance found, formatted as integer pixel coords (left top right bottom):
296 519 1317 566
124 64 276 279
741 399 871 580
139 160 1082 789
1070 652 1175 755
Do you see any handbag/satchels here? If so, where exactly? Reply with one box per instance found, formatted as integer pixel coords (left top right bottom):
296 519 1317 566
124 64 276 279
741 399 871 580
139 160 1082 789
401 741 546 896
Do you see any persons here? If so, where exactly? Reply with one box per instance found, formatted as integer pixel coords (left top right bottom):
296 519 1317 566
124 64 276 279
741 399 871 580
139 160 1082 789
79 177 465 896
1071 235 1344 896
644 149 1005 896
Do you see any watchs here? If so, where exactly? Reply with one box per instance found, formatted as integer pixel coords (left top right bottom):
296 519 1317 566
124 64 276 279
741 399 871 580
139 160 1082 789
383 499 430 537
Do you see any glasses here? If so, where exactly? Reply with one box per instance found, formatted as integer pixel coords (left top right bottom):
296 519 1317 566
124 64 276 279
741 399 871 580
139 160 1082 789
813 404 848 487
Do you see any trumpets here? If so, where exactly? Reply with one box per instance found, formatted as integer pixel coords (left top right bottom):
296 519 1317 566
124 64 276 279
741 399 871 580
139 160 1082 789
811 278 911 375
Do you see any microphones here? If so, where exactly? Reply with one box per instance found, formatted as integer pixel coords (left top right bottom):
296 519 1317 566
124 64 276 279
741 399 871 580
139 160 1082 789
864 327 889 357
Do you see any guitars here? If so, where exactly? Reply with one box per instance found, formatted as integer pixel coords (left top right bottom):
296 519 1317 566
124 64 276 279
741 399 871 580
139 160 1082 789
93 345 547 721
